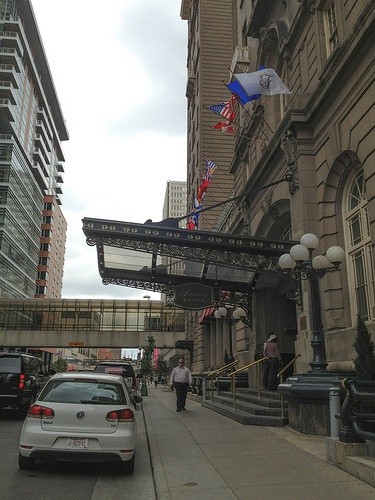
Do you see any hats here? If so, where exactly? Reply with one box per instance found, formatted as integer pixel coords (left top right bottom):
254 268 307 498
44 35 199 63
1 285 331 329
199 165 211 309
268 335 276 341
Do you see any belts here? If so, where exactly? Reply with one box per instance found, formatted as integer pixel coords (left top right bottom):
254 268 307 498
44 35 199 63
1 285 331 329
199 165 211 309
176 382 187 384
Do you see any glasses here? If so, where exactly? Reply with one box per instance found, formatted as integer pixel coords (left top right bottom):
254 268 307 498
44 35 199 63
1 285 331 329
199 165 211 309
178 361 185 364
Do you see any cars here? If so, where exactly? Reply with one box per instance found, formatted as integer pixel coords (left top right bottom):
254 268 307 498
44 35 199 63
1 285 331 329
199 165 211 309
18 361 143 473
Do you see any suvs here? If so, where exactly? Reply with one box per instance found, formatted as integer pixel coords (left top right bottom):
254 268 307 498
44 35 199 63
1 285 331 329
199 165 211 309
0 352 56 420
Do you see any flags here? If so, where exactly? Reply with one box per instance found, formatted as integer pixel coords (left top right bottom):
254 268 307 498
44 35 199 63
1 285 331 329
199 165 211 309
205 158 217 183
207 93 240 122
187 176 213 230
233 68 291 99
227 66 267 105
212 121 234 134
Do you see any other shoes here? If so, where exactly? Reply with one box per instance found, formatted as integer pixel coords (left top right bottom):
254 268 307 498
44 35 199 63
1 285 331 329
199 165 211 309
176 407 181 412
182 407 186 410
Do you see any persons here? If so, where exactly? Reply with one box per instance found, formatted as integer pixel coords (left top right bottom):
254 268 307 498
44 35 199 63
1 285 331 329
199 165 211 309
170 359 192 412
262 333 281 390
147 374 165 388
